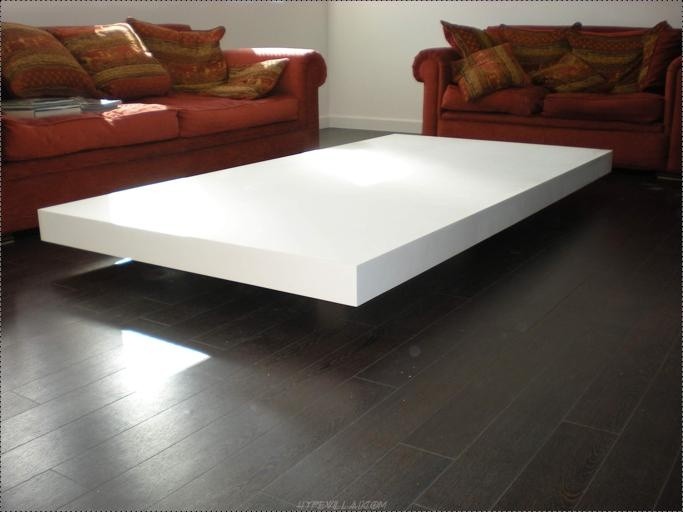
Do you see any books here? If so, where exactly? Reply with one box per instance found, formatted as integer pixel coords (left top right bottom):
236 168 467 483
1 93 121 119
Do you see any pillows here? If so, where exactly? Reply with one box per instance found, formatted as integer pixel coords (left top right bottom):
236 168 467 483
439 20 674 104
0 15 291 102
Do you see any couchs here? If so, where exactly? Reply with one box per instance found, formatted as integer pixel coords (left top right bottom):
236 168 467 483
0 24 329 248
411 25 682 174
